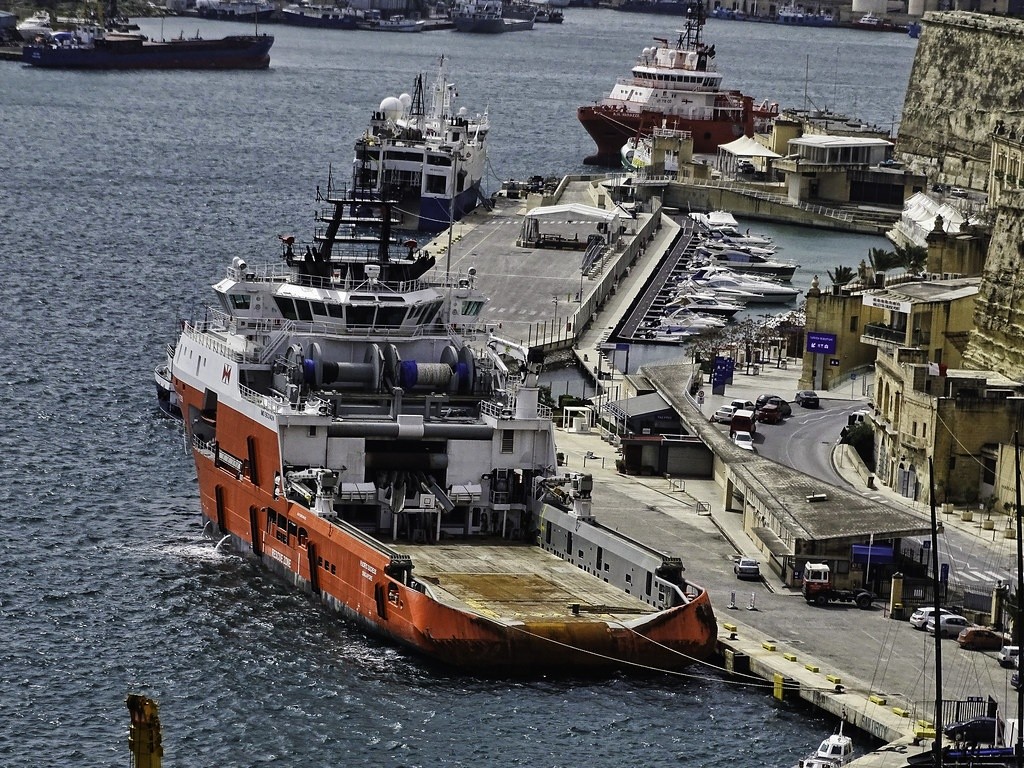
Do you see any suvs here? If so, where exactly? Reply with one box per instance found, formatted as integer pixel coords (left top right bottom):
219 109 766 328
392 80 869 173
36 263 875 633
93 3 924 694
729 407 757 438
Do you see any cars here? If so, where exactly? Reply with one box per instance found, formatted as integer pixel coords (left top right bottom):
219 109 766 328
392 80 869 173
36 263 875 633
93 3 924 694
714 405 738 425
730 393 793 424
997 645 1019 692
795 390 819 409
732 430 756 453
925 614 978 638
909 607 956 631
956 627 1011 651
733 557 761 581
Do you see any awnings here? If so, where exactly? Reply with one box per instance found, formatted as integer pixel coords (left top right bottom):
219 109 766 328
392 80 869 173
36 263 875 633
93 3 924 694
602 392 671 420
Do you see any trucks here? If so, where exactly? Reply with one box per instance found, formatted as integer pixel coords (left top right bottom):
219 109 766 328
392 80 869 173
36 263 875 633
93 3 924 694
802 561 878 611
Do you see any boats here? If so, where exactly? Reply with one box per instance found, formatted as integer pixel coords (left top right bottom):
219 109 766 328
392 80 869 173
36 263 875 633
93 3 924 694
143 137 806 694
15 1 573 72
793 718 856 768
577 0 898 172
342 54 488 238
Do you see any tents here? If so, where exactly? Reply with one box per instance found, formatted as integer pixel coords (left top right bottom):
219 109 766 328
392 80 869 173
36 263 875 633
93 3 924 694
516 203 621 248
716 134 782 182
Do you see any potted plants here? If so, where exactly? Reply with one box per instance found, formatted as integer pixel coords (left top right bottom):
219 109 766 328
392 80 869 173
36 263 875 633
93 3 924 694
938 478 954 513
959 486 980 521
980 494 1000 529
1002 502 1023 537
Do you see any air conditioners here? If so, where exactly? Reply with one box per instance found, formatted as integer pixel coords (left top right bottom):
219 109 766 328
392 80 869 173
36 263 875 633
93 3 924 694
953 272 962 279
922 272 931 281
943 273 952 280
931 273 942 281
